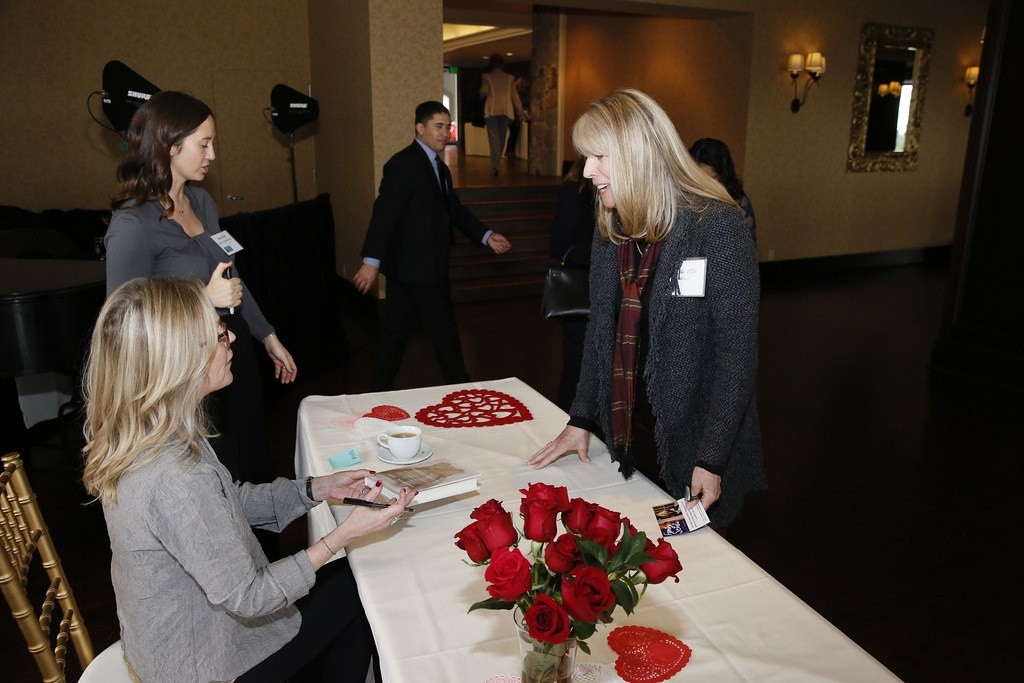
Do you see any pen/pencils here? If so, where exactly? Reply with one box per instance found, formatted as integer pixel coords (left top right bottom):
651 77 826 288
226 267 234 315
343 498 415 512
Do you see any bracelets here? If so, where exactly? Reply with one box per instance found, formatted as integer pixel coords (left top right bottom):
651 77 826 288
321 538 336 555
306 476 323 502
262 331 275 343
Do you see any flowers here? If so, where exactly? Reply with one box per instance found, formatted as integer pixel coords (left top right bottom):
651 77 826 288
455 480 684 680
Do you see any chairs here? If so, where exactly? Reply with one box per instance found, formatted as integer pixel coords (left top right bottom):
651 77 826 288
0 453 140 683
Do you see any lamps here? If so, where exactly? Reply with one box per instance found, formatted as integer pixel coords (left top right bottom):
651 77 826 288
787 52 826 113
877 81 902 98
964 65 980 116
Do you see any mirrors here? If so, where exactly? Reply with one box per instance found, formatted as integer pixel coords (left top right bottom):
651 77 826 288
845 23 935 171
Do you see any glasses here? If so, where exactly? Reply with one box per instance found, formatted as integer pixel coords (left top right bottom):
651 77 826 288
200 322 231 348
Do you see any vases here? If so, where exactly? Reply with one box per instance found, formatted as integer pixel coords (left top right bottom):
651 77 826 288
512 598 580 683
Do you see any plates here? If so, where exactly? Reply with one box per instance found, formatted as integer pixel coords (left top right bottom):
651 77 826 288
377 442 433 465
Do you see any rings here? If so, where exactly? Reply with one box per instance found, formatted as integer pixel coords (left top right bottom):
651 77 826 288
547 441 553 446
389 516 401 527
282 366 286 369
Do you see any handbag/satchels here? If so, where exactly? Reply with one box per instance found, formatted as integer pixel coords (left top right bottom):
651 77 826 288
541 245 592 318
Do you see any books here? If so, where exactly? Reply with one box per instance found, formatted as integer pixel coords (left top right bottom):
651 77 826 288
364 457 482 508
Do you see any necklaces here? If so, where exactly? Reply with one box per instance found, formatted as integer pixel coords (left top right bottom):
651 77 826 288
167 196 186 214
635 240 643 256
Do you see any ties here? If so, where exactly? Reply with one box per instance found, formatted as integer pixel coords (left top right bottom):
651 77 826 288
435 154 447 196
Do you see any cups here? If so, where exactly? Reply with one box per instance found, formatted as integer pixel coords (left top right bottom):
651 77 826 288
377 426 422 459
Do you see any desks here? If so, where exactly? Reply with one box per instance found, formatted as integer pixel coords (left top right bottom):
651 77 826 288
294 377 907 682
0 257 108 381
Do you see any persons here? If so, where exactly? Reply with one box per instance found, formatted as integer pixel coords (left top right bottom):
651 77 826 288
353 101 512 391
81 277 419 683
689 138 757 244
479 53 527 176
527 88 773 556
504 78 526 159
104 91 297 563
540 152 595 431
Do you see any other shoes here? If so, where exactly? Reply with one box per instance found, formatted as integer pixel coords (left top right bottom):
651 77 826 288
492 169 500 176
